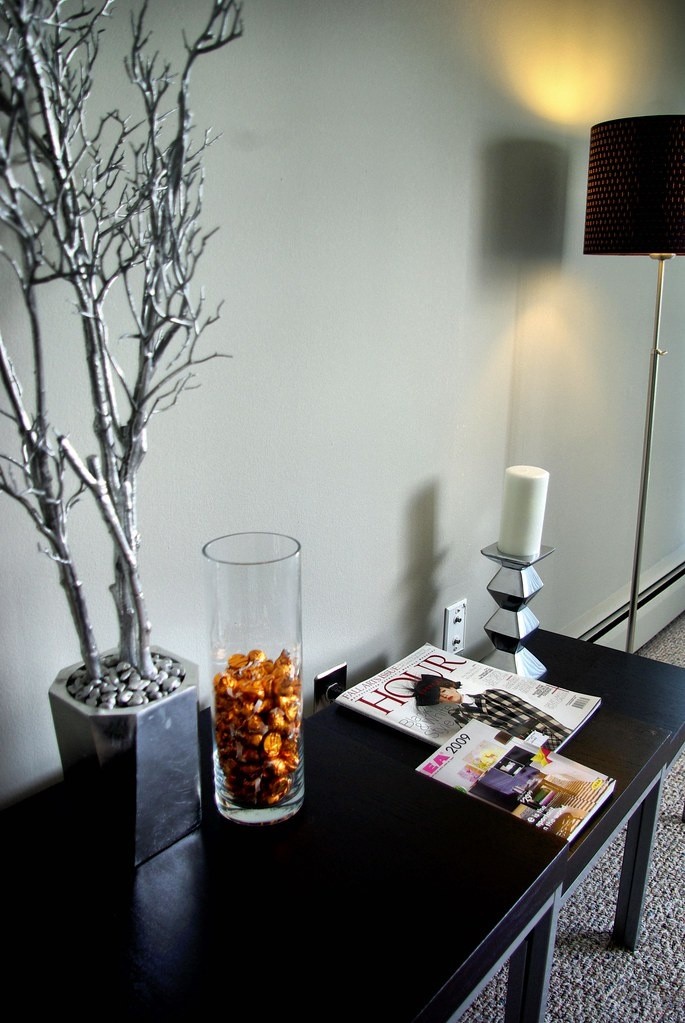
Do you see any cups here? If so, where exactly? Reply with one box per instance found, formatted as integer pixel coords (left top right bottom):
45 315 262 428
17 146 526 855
201 531 305 823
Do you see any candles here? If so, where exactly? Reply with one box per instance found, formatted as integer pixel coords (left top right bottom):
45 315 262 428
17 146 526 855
497 464 550 560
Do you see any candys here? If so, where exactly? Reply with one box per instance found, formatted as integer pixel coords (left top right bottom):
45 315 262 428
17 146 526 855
212 648 301 806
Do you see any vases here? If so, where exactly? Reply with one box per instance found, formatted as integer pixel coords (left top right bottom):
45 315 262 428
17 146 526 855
48 644 202 869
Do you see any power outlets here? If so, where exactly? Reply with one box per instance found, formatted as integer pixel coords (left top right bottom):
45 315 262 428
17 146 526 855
314 662 348 713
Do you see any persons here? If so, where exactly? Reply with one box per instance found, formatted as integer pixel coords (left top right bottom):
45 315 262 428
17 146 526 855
414 674 573 751
334 643 602 753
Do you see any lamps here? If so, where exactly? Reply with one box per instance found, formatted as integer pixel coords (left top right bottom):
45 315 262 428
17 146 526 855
582 115 684 654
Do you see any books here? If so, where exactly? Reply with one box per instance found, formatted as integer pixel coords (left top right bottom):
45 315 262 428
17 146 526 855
415 718 616 844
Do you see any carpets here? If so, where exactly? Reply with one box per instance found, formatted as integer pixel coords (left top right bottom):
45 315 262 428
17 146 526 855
458 610 684 1023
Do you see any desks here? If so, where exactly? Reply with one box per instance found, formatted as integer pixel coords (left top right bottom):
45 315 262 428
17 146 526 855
0 629 684 1023
